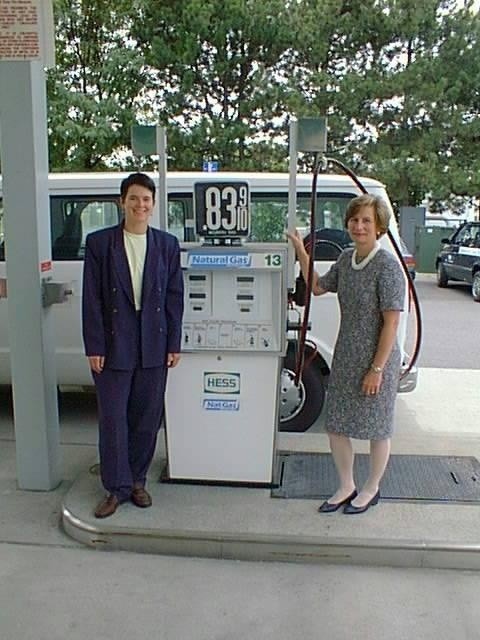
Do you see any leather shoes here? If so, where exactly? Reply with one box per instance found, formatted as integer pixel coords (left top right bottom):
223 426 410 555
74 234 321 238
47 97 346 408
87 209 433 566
94 487 153 519
317 488 381 515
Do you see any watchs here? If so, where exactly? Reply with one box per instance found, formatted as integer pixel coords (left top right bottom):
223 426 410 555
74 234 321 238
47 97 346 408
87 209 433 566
369 363 384 376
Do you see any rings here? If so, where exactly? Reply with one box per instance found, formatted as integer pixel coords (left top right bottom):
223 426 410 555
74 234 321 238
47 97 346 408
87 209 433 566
370 391 377 395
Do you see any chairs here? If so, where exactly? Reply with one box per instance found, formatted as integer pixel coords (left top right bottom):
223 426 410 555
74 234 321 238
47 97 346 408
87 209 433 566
302 228 352 260
52 215 82 260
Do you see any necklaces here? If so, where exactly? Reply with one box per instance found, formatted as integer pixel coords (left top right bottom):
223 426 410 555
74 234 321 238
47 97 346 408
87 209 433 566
349 239 382 273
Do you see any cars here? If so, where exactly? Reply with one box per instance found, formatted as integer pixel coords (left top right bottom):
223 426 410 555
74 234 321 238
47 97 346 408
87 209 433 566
436 222 480 302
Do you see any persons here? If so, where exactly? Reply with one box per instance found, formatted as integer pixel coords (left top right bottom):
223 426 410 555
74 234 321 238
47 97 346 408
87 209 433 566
81 171 186 518
283 194 407 514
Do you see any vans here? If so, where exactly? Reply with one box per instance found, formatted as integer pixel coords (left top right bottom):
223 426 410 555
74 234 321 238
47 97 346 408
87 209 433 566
0 170 419 432
